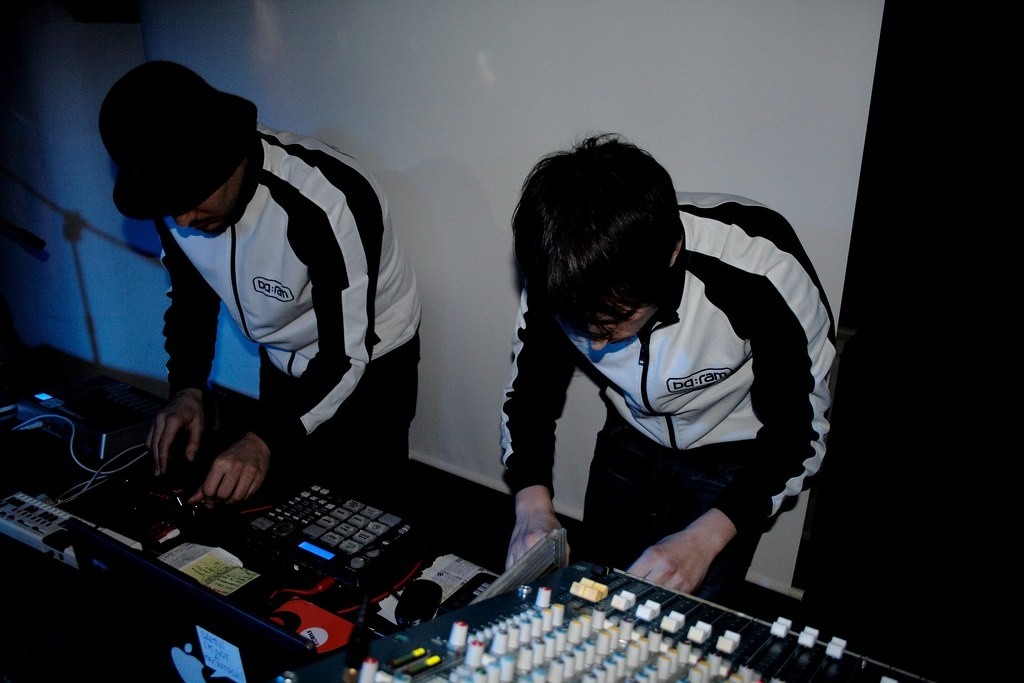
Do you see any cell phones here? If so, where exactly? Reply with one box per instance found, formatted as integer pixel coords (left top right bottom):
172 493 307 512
438 573 498 613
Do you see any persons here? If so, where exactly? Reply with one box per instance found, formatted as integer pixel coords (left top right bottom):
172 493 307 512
498 135 836 596
98 60 424 507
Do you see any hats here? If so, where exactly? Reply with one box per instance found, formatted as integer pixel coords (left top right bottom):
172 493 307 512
98 60 259 219
